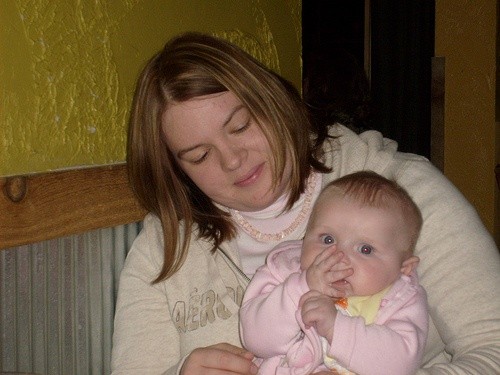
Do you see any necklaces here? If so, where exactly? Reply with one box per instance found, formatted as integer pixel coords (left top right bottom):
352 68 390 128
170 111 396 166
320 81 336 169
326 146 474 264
231 164 316 244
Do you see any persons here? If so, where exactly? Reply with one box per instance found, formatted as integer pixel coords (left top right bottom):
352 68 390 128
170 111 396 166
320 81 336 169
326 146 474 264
108 32 499 373
237 168 431 374
